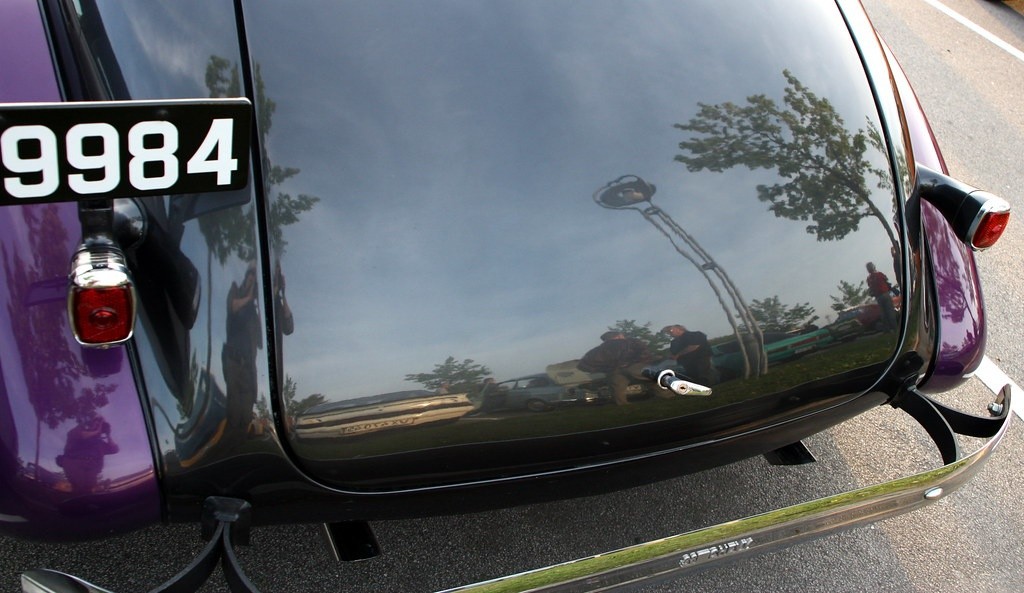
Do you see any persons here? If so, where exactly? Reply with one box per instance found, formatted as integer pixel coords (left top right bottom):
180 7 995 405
222 259 294 412
61 410 119 493
577 324 715 406
440 381 449 394
866 262 898 330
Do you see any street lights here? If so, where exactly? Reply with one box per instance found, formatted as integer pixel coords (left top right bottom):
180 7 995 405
591 174 769 382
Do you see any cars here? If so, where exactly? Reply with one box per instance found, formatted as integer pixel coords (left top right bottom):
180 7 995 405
711 329 832 382
823 296 902 343
482 371 601 414
296 389 478 446
